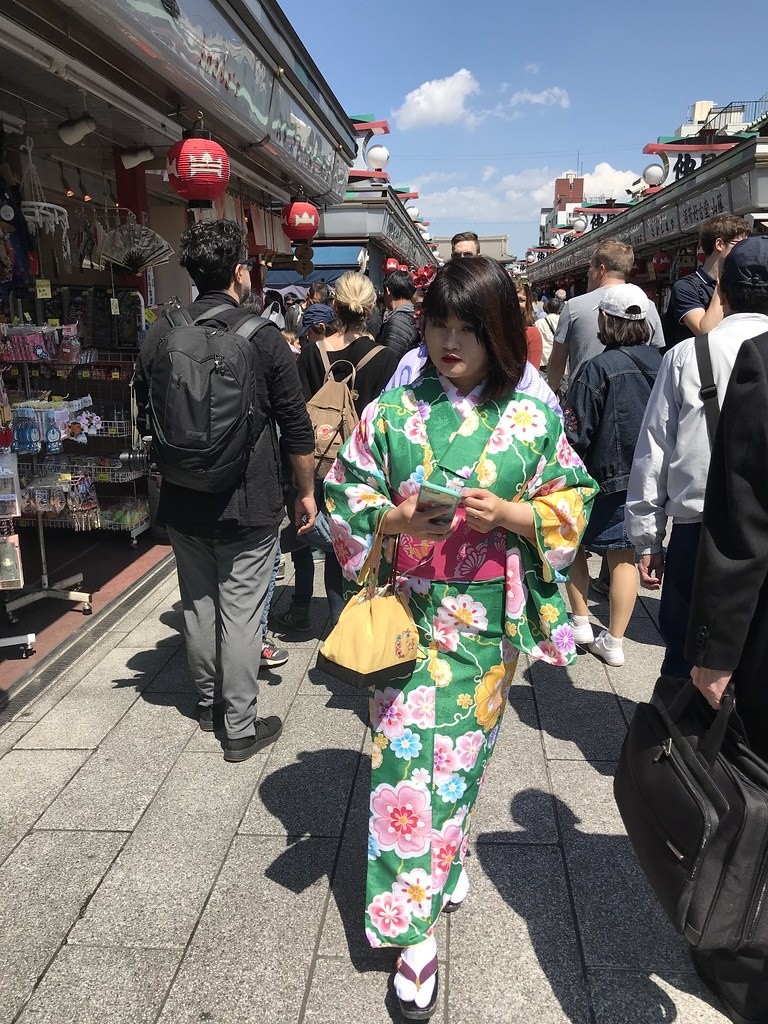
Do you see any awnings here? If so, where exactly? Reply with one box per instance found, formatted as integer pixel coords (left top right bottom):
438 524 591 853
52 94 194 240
263 246 370 290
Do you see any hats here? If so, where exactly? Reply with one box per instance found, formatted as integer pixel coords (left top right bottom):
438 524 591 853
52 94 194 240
294 304 334 338
593 283 649 319
721 235 768 286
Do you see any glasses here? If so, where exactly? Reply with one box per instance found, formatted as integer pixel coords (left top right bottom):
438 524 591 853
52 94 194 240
451 251 478 259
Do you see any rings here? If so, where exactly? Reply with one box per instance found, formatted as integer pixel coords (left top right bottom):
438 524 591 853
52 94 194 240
473 510 479 519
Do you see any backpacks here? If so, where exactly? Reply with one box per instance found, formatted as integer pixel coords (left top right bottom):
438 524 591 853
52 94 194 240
145 304 282 493
292 340 388 490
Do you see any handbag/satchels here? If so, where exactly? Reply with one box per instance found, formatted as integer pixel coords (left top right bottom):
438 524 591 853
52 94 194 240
676 247 696 277
613 673 768 953
315 506 420 689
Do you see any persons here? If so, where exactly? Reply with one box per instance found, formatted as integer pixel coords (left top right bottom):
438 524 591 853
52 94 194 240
319 251 601 1019
237 267 568 667
134 217 283 763
624 210 768 1024
450 232 484 259
556 282 664 666
547 237 665 600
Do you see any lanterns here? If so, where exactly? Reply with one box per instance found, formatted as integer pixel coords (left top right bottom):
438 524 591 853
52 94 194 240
281 189 320 247
381 258 399 274
398 265 409 273
652 248 671 272
697 241 706 263
629 256 646 276
165 118 231 225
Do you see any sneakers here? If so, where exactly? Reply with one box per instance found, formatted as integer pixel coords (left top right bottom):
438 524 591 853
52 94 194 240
276 554 286 580
591 578 609 600
260 638 289 665
569 620 594 644
224 716 283 762
588 630 625 666
199 704 224 730
277 603 313 631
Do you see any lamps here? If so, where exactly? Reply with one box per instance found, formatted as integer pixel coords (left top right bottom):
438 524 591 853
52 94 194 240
76 167 91 201
242 171 290 205
573 219 585 233
259 189 269 265
107 179 119 207
433 252 444 267
163 126 245 179
0 14 58 73
57 62 165 134
267 194 276 267
58 161 75 197
527 255 534 262
119 124 154 169
367 144 391 171
422 232 430 242
407 206 418 221
642 163 663 189
0 111 26 135
58 88 97 145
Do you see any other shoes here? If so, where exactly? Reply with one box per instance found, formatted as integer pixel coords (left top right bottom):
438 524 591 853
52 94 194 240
312 549 326 564
693 955 757 1024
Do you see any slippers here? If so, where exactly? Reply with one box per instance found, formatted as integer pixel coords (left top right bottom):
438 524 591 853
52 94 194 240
441 900 461 912
393 954 439 1019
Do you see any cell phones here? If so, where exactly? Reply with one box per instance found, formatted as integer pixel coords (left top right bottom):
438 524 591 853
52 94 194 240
415 482 461 527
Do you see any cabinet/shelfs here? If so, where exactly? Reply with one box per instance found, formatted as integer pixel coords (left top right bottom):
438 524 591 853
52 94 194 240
0 293 153 663
